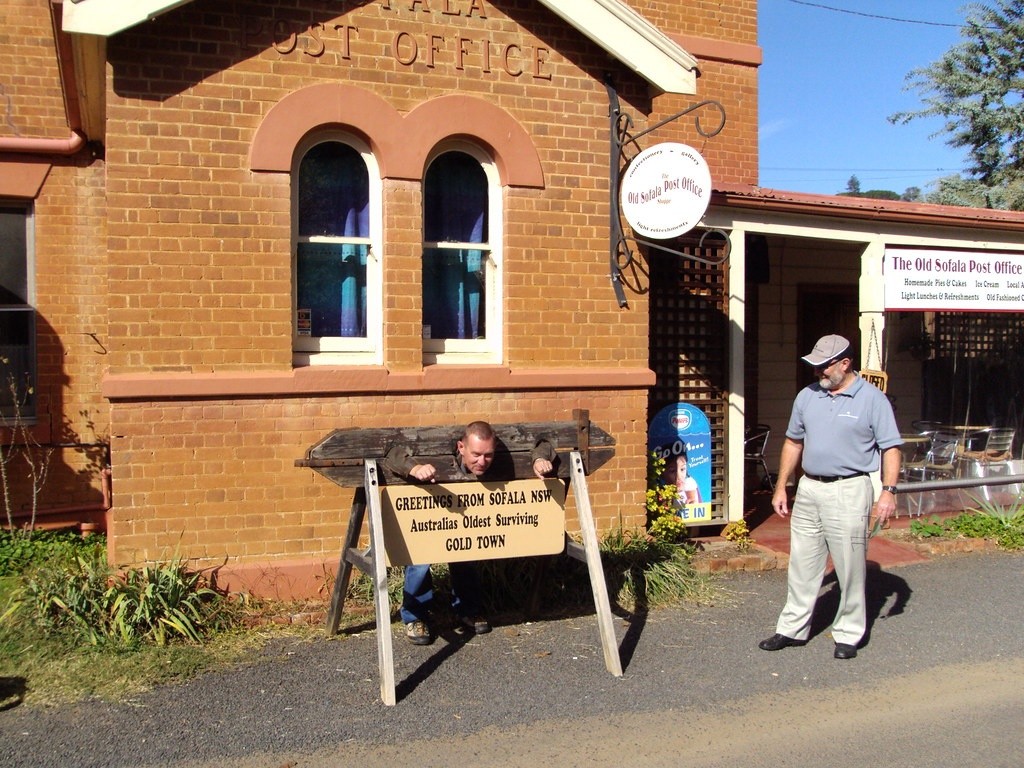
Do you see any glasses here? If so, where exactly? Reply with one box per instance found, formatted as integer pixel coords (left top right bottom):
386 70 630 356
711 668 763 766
821 359 842 368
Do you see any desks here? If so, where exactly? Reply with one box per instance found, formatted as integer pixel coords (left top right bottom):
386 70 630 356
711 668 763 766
894 433 930 520
939 425 992 489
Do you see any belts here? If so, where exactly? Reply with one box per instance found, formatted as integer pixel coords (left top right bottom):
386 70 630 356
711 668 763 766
805 473 863 483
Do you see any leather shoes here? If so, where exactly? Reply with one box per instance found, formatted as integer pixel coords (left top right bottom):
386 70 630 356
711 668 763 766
834 644 857 658
759 634 806 650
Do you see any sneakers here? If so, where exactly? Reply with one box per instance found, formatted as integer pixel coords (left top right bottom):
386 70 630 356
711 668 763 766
456 614 490 634
407 620 431 645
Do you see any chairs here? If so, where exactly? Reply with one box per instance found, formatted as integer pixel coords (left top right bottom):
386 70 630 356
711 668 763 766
744 423 774 492
899 419 1015 518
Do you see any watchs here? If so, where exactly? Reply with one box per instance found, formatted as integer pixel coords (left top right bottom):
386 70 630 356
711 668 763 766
883 486 898 495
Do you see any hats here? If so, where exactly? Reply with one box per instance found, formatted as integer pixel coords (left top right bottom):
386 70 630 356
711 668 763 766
801 334 850 366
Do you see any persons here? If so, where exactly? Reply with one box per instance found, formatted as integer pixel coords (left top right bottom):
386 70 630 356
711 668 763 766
760 332 904 659
655 437 702 508
384 421 558 645
